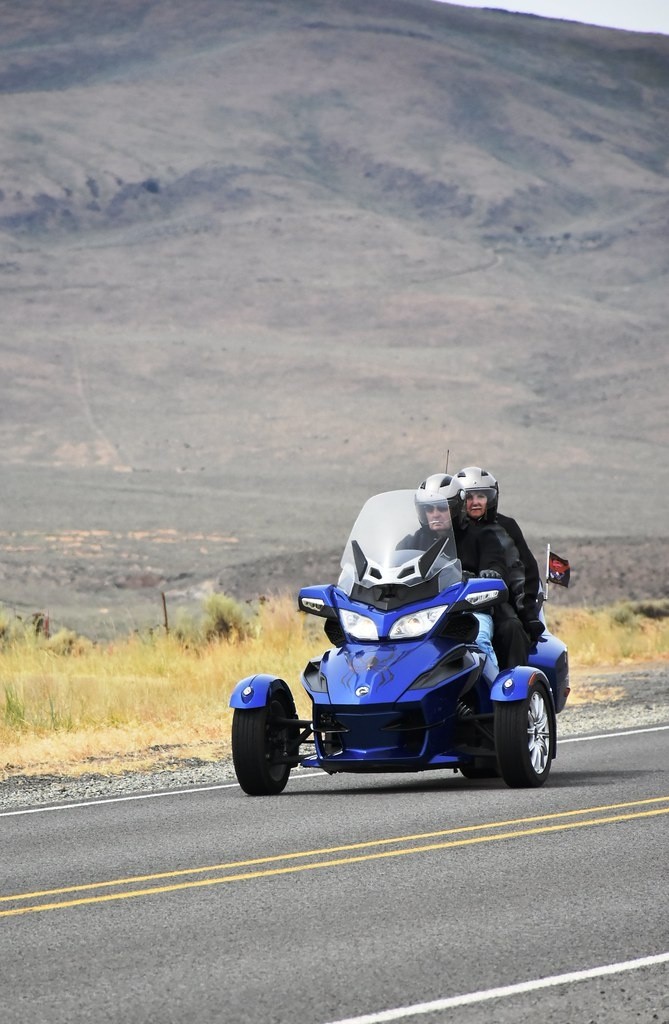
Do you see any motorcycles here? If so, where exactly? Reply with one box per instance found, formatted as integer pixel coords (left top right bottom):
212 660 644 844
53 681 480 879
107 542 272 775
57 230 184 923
229 489 570 795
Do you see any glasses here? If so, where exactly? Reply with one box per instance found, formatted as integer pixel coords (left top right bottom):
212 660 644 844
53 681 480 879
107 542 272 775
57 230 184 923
421 504 450 513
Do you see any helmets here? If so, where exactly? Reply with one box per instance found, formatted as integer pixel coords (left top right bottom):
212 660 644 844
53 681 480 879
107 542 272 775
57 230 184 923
454 467 499 517
416 473 467 530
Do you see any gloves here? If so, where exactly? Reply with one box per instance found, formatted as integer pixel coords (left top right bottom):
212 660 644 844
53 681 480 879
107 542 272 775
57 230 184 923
480 570 502 579
527 620 545 640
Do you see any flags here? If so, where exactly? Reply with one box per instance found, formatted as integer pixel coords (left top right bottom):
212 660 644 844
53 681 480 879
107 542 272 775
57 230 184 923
548 552 570 588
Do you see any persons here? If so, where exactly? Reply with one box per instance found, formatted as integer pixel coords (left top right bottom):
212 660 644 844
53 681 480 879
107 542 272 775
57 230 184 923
396 467 545 671
